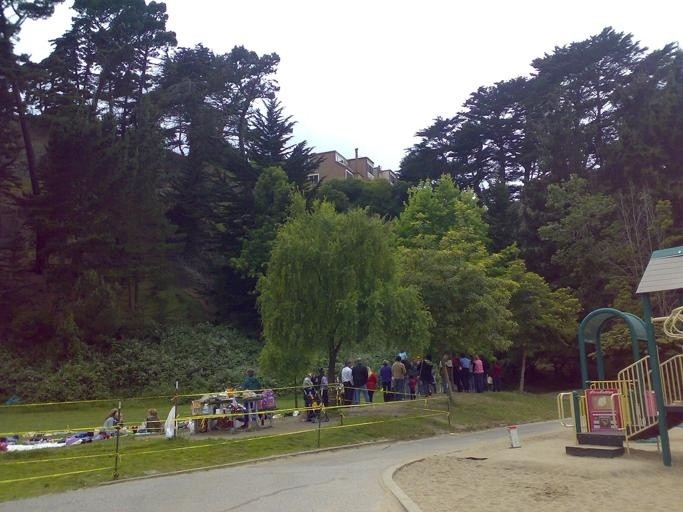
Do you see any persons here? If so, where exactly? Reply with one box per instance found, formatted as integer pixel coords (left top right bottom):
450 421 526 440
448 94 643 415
103 408 123 430
302 350 502 424
131 408 161 435
239 368 263 429
